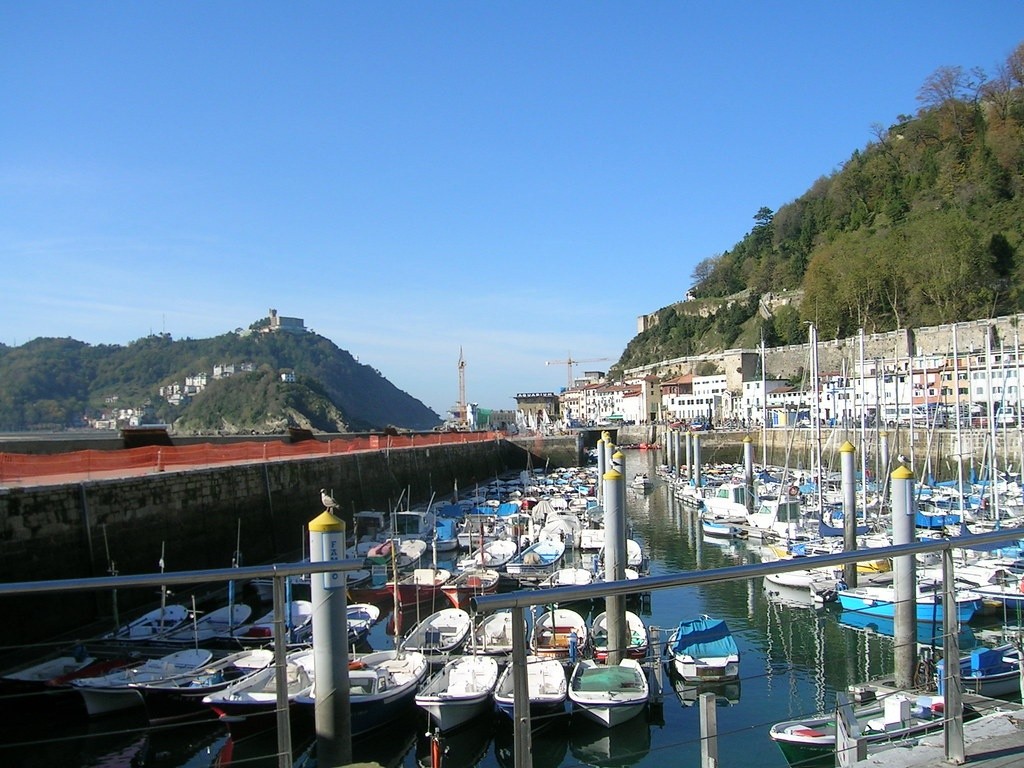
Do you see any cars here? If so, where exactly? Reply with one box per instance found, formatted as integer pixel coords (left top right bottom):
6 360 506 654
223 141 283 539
997 406 1016 427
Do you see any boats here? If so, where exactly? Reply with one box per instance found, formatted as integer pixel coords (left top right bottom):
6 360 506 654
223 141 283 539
492 656 568 721
0 647 428 744
568 656 650 728
590 610 647 662
414 654 499 734
399 608 472 672
666 617 740 682
769 625 1024 768
838 608 980 656
462 610 528 672
665 660 741 707
529 608 588 667
415 709 651 768
100 600 381 650
250 468 653 610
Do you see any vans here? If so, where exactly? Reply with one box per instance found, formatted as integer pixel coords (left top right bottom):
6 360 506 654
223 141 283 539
880 404 933 428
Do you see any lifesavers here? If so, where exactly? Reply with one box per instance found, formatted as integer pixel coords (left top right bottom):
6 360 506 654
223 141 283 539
789 485 799 496
865 470 871 478
349 662 366 670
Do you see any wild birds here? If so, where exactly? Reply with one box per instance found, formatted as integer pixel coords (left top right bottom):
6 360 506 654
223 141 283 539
319 488 342 511
609 458 623 469
897 454 911 466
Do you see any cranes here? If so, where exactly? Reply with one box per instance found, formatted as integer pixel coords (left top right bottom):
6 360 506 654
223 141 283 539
546 357 610 390
457 346 467 423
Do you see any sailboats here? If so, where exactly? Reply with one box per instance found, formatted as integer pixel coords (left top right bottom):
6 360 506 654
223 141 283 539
655 321 1024 625
